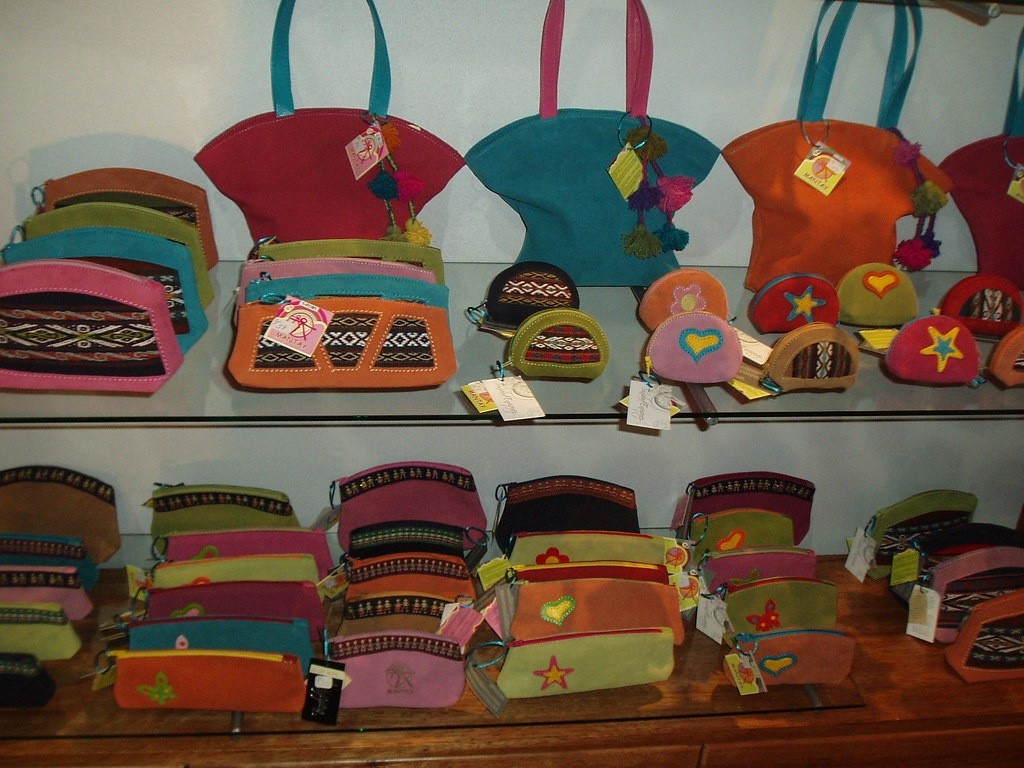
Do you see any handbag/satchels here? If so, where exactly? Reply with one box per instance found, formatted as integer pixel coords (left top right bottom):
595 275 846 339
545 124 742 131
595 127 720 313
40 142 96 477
721 0 953 294
937 26 1024 291
464 0 721 286
193 0 466 249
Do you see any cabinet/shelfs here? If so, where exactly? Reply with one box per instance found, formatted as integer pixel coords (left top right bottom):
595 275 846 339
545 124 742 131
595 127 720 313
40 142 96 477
0 256 1023 768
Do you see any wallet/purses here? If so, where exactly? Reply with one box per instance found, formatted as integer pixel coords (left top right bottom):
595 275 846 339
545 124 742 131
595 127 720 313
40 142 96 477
486 260 1024 394
0 167 461 396
865 489 1024 684
0 464 858 716
